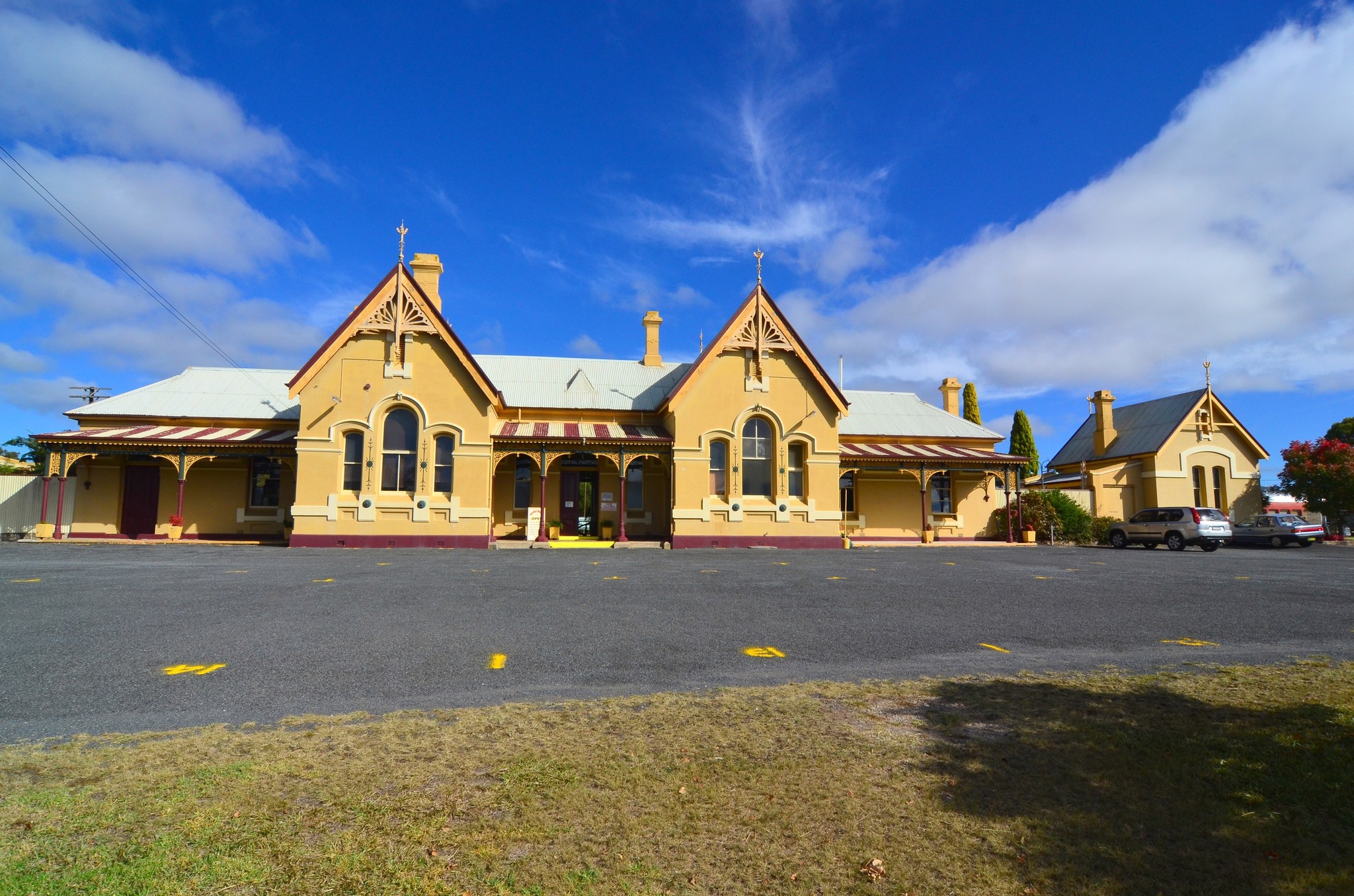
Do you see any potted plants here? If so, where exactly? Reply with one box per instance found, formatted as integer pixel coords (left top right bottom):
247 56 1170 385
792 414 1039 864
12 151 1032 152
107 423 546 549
284 519 293 539
545 520 563 538
922 524 934 541
35 521 55 538
599 519 617 538
1023 524 1036 542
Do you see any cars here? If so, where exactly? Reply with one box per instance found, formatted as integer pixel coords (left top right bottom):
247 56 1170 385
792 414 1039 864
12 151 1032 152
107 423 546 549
1222 513 1326 548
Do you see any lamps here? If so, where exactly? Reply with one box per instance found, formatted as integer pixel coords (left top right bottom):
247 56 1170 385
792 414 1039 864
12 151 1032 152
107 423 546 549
332 396 341 403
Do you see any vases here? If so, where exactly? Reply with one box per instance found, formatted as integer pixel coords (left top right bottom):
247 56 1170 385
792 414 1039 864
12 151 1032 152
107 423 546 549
167 526 184 538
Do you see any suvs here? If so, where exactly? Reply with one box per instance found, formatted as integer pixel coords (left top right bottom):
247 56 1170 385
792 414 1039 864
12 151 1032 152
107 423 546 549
1107 506 1233 551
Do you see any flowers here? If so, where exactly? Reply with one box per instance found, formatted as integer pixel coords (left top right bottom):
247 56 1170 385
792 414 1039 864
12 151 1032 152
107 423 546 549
169 514 185 527
